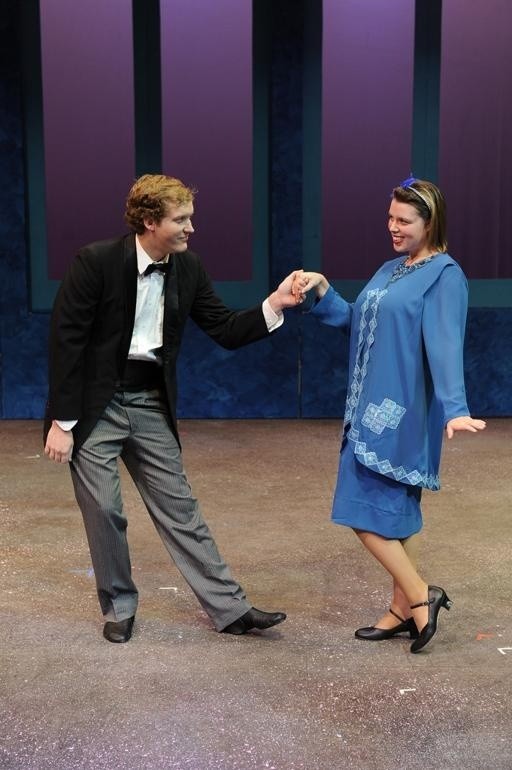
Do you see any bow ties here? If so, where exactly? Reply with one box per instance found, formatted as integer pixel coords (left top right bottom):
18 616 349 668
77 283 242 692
144 263 172 276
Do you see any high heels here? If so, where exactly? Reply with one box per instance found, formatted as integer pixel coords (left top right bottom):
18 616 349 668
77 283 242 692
355 585 454 653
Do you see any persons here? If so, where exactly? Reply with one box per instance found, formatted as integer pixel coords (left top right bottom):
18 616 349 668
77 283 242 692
42 173 309 644
291 177 487 653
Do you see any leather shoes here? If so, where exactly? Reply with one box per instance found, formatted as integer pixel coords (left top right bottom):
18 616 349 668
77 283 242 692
224 607 286 635
103 616 134 643
